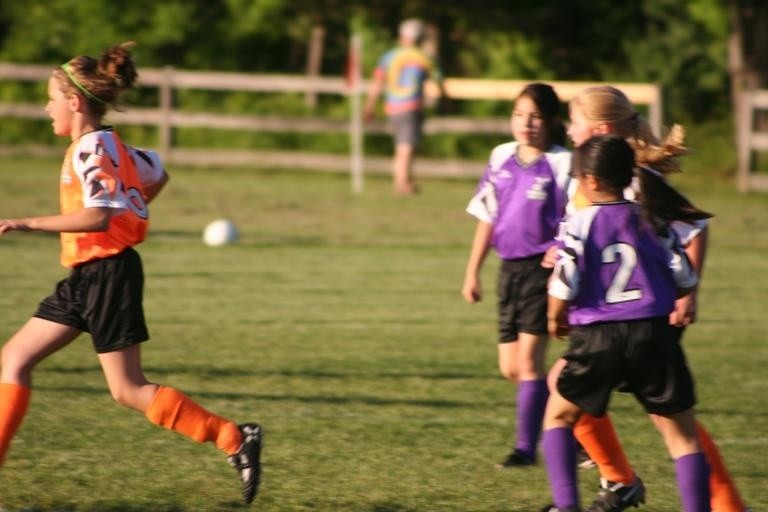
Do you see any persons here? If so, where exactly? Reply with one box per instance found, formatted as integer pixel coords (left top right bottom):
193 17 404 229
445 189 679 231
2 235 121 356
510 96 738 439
362 15 448 195
462 84 747 510
0 44 263 507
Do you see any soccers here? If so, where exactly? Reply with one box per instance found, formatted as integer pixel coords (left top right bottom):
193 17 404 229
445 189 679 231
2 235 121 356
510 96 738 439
202 219 240 247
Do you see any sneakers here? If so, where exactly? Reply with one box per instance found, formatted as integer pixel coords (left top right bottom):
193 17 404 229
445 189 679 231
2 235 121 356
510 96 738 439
533 477 647 512
226 422 263 505
497 447 536 466
